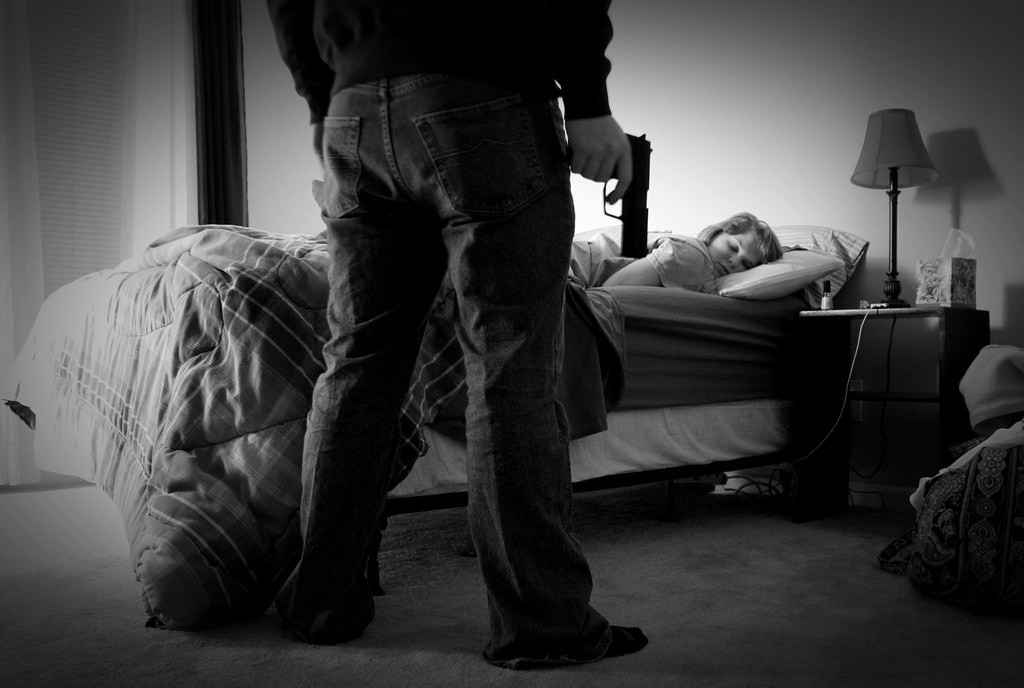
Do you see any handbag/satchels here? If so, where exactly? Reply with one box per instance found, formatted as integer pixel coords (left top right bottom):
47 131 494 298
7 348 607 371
877 445 1024 614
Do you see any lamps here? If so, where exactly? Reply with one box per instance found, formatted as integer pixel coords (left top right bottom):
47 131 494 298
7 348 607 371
852 108 942 307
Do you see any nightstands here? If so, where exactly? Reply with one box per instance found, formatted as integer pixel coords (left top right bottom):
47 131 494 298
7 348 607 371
786 309 990 523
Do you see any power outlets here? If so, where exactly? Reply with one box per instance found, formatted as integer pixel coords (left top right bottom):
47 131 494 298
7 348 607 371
848 380 863 421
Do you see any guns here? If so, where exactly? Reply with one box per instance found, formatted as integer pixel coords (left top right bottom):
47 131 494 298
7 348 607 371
567 129 652 262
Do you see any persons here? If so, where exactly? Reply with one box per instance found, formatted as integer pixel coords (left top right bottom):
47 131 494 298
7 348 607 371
573 212 784 295
268 0 650 672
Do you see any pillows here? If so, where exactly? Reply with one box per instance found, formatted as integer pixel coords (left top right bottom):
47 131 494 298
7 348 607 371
775 225 870 309
715 250 847 299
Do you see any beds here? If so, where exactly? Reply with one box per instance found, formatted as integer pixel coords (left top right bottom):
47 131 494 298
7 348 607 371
2 223 841 632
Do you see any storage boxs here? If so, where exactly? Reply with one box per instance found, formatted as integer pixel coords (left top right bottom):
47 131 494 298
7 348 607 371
915 258 976 310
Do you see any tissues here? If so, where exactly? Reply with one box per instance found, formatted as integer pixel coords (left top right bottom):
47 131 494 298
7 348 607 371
915 228 977 307
